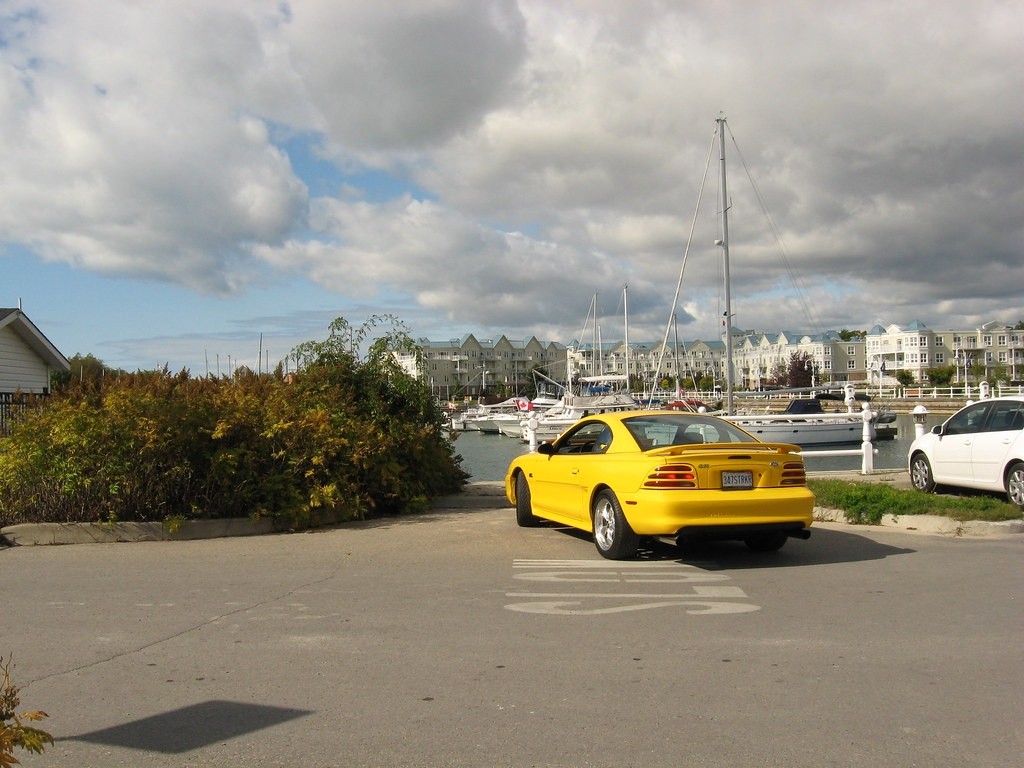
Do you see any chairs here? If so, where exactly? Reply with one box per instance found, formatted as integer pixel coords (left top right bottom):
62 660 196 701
672 433 703 446
1004 411 1022 427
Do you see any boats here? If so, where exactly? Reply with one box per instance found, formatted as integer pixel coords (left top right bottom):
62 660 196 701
436 109 900 448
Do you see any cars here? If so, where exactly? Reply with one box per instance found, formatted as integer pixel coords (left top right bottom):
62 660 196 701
907 394 1024 509
502 408 817 561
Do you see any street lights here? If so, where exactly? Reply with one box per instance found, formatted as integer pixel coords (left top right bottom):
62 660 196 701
705 366 716 396
637 371 646 397
954 347 967 395
803 359 816 399
